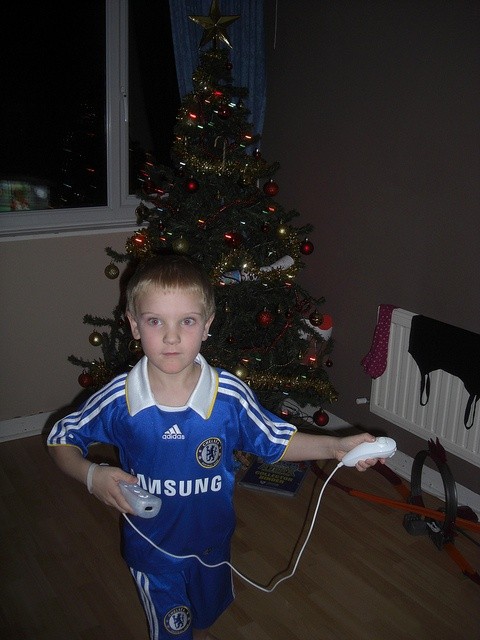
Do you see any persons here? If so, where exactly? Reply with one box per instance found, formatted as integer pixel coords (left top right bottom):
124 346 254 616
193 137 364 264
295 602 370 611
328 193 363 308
44 246 386 640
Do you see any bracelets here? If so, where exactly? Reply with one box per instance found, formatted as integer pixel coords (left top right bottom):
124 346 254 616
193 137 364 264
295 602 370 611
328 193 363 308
86 461 99 494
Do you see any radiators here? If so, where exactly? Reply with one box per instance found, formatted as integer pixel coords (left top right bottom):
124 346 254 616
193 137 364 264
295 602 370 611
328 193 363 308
369 304 480 469
